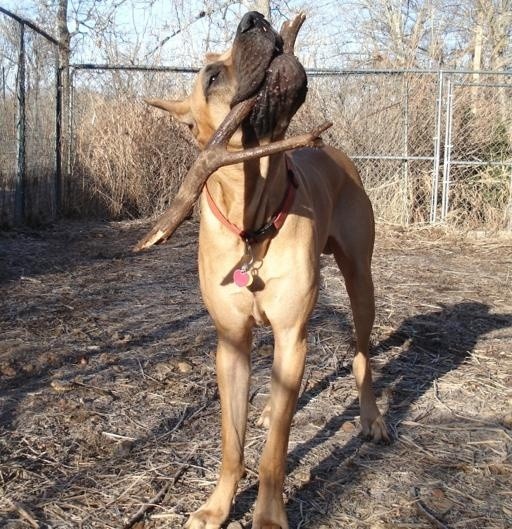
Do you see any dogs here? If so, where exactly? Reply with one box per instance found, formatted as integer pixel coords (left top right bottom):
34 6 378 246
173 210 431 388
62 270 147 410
144 10 394 529
